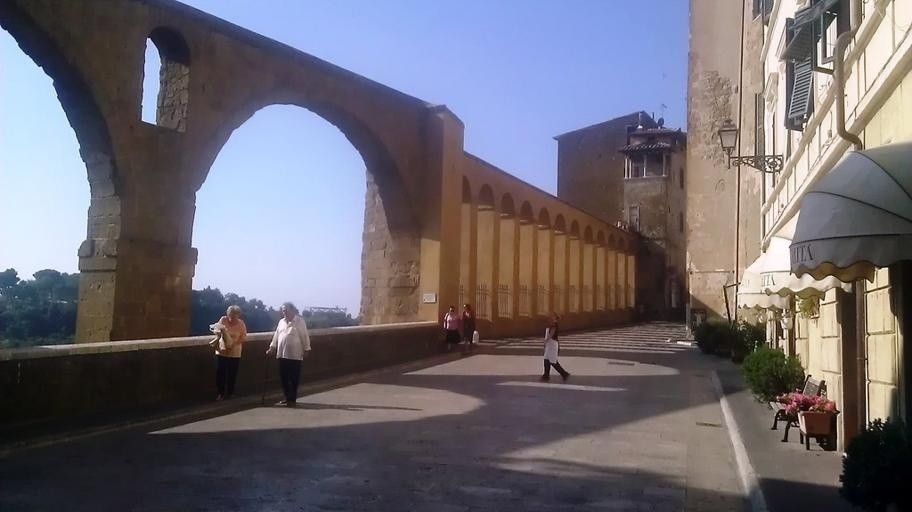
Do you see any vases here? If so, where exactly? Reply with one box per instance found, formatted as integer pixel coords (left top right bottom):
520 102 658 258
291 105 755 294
796 410 832 435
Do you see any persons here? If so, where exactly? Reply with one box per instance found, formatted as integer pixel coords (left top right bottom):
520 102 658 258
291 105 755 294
266 302 312 407
213 305 248 402
461 304 476 356
444 305 461 352
540 310 570 381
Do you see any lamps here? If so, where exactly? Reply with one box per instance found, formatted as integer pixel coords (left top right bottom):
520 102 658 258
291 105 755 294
779 318 793 330
718 117 784 173
758 314 768 323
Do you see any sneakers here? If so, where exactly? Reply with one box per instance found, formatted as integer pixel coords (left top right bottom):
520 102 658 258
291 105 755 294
280 401 295 408
446 348 474 357
214 393 236 402
540 371 570 384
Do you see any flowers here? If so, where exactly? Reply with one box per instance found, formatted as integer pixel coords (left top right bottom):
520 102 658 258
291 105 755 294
776 392 839 414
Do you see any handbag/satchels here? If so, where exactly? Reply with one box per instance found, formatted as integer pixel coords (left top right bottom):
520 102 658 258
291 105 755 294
438 327 448 341
472 330 480 345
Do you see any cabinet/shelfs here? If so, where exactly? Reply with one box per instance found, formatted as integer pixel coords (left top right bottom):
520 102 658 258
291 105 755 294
798 429 832 450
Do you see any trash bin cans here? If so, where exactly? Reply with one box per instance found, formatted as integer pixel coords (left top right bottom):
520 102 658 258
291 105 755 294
691 308 707 335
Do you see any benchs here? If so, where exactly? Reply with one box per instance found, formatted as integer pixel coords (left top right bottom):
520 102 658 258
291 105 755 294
769 374 826 442
